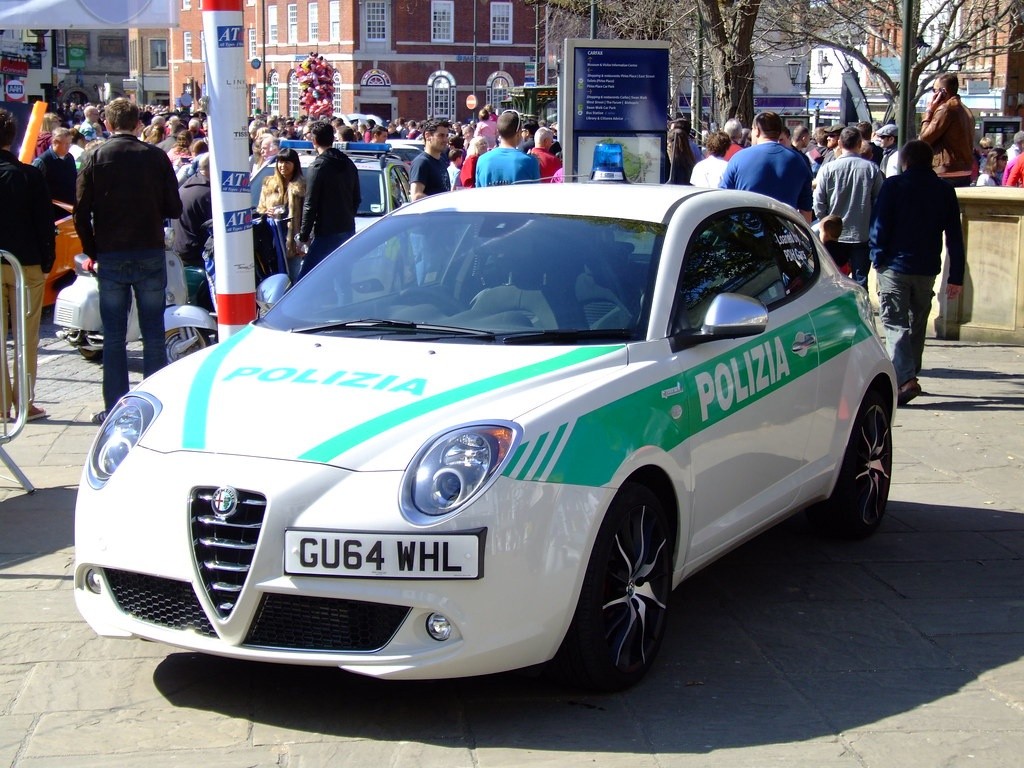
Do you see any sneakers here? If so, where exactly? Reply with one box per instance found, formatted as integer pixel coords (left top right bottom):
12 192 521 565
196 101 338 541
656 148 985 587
91 411 107 424
15 405 47 420
2 411 11 423
899 378 922 406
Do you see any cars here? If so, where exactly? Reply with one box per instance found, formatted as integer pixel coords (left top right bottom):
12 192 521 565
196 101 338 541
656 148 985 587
72 143 899 699
250 139 417 303
382 138 427 168
17 101 83 308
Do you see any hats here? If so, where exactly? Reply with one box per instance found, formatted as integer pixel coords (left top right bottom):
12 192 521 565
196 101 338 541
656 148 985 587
366 119 376 130
823 124 846 133
876 124 898 137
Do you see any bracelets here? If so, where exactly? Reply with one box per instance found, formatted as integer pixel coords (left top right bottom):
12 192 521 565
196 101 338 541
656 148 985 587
925 109 933 112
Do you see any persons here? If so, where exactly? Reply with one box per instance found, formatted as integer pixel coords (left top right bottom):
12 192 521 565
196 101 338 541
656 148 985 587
0 109 55 422
295 122 361 283
256 148 306 289
717 112 812 237
170 152 219 347
970 130 1024 189
249 104 565 188
917 73 975 187
869 139 965 406
72 98 183 424
818 214 848 276
667 112 899 189
408 119 451 285
812 126 884 292
29 98 208 226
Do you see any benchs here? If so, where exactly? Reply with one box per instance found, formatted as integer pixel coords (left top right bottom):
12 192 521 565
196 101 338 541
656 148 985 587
558 242 647 329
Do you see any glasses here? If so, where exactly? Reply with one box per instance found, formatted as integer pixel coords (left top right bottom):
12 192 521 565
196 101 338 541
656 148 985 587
501 109 520 132
981 146 994 149
999 155 1009 161
932 87 939 92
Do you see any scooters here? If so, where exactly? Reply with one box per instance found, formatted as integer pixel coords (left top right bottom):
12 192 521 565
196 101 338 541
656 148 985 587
53 204 291 368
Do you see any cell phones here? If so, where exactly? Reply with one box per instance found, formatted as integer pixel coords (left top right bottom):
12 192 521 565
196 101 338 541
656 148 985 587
935 88 947 104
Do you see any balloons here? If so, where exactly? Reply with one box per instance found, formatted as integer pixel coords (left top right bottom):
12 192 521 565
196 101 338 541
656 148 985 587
296 51 335 118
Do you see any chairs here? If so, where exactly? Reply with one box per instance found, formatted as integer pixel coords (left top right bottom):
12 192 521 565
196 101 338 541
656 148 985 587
468 247 557 330
681 236 716 283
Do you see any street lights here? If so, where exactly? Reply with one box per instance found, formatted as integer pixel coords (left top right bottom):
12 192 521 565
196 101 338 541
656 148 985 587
785 51 833 116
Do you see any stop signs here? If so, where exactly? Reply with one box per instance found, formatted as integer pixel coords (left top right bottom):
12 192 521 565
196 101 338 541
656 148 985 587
466 95 476 110
